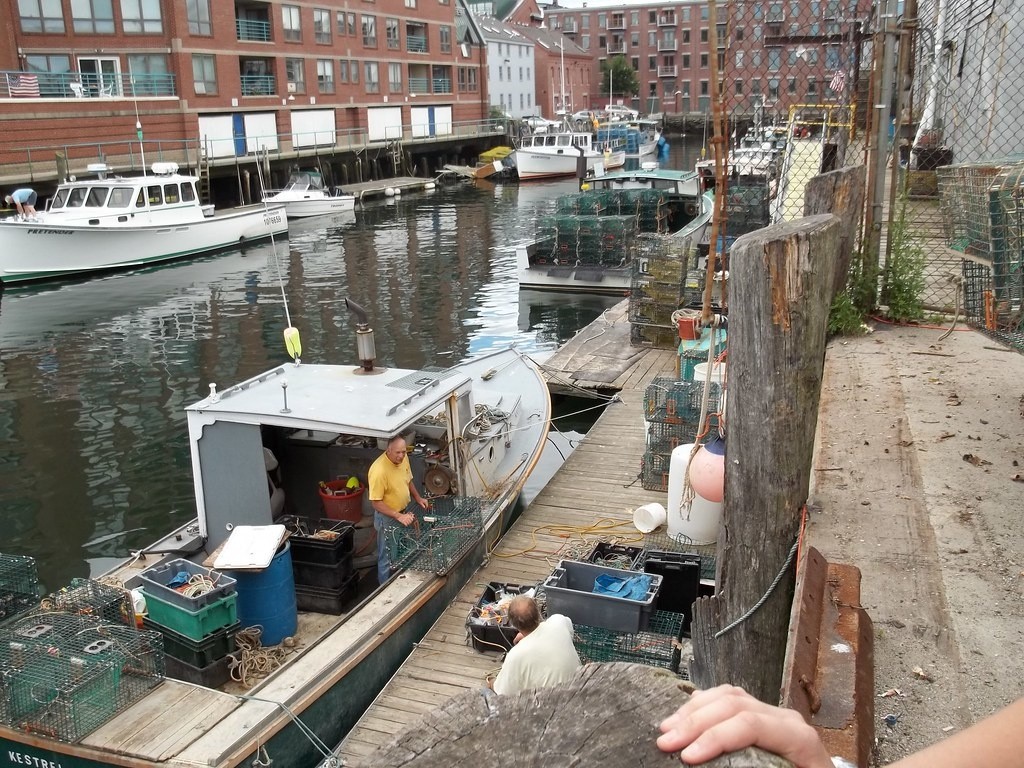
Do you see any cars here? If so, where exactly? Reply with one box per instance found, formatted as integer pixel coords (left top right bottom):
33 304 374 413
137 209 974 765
571 110 615 125
522 116 558 132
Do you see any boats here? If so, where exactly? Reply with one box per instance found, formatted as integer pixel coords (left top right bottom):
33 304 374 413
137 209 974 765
697 126 790 199
1 347 554 766
0 162 288 291
260 171 356 217
515 168 715 299
598 120 661 158
506 132 626 181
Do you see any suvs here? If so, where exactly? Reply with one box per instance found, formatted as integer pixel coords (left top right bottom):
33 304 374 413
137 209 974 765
604 104 639 118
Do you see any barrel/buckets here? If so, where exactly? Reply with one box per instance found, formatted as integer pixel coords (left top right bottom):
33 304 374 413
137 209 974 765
217 539 298 648
320 480 364 523
693 361 727 412
666 444 721 546
121 602 148 629
632 503 666 534
716 236 734 254
678 317 702 340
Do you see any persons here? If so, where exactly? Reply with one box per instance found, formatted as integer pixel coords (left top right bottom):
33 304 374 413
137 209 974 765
70 192 83 206
367 434 429 586
588 111 600 140
728 172 739 186
487 594 584 694
5 188 39 223
655 644 1024 767
702 169 715 191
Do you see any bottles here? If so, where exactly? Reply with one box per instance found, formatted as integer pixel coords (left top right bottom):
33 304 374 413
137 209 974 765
319 481 334 496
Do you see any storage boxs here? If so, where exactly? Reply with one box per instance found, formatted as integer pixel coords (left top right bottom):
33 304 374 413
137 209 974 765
0 101 1024 767
